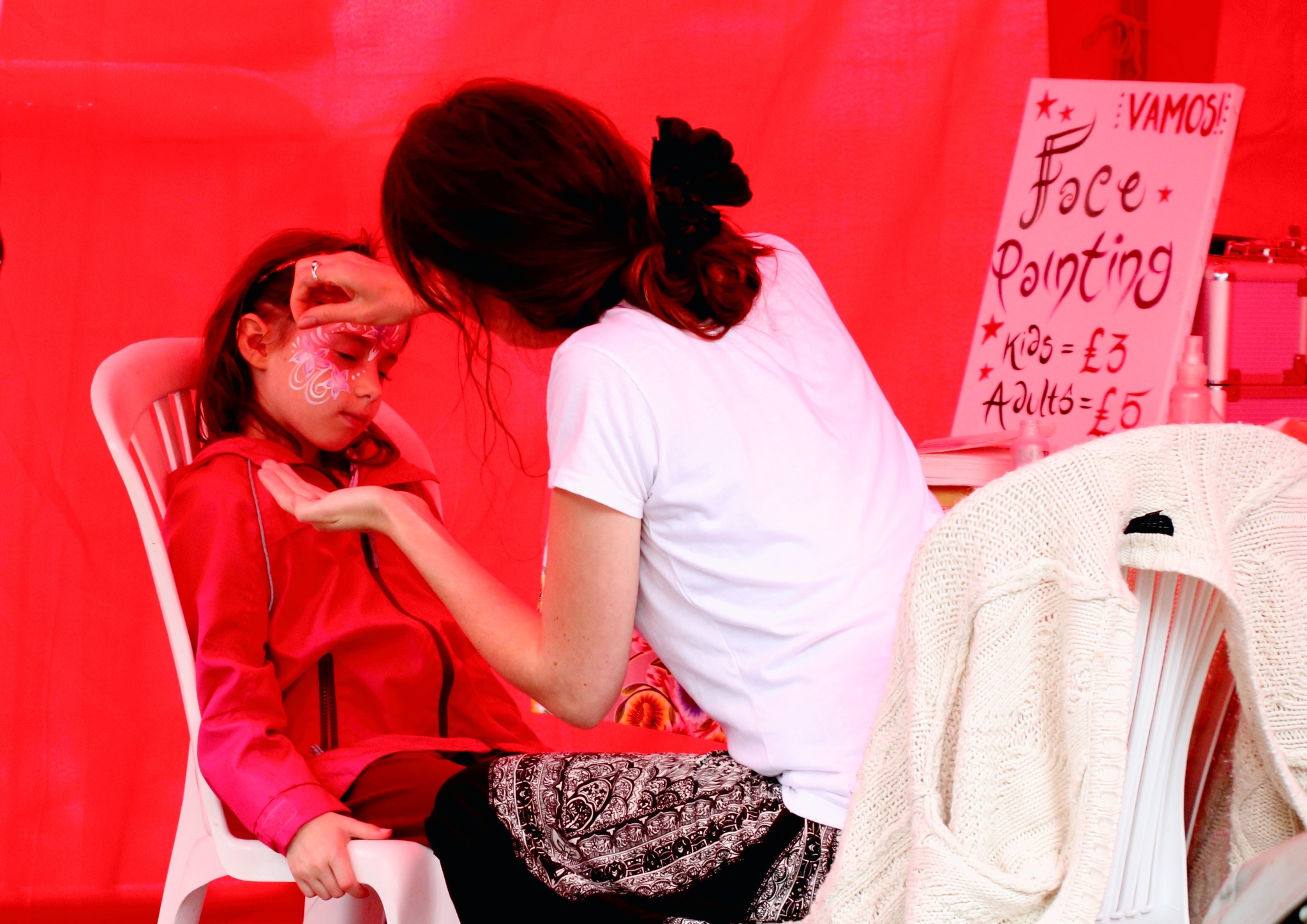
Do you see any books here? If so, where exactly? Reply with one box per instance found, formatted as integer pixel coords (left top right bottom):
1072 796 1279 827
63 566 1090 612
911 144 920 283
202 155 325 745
916 433 1054 485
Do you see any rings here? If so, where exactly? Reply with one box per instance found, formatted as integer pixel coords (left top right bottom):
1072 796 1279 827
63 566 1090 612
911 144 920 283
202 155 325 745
310 260 323 280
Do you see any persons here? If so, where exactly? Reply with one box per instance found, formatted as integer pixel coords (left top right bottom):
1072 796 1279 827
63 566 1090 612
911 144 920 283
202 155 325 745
159 228 547 901
259 78 944 924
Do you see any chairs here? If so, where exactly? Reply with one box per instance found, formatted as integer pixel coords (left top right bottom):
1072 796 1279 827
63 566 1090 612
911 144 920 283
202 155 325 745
801 426 1307 923
89 337 463 924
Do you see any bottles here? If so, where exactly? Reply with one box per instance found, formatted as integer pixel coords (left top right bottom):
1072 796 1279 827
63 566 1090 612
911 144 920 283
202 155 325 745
1012 420 1048 473
1170 336 1210 425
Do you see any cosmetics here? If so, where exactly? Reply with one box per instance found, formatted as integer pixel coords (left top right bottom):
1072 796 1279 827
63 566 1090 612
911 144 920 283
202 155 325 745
1011 419 1049 470
1169 337 1210 425
